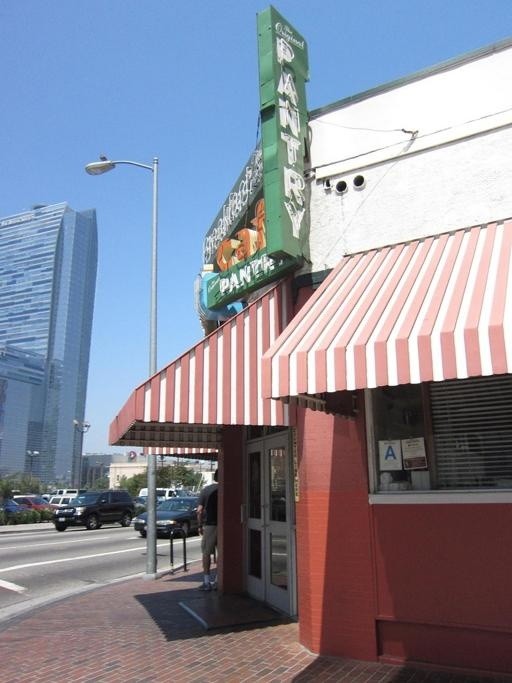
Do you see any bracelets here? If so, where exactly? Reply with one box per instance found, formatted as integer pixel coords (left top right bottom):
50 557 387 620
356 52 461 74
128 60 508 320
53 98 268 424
197 524 202 527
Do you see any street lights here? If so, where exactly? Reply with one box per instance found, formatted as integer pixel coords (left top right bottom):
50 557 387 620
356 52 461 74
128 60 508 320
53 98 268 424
72 419 90 489
84 155 163 580
26 450 40 482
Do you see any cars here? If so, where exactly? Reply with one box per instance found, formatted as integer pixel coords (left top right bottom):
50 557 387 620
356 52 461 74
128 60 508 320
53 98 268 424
134 496 200 539
272 494 286 518
0 486 193 521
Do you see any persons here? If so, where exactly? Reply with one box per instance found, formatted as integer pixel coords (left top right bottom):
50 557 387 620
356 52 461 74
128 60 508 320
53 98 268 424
196 467 218 593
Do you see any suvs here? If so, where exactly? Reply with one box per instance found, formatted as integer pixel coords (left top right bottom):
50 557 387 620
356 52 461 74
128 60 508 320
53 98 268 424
51 490 136 531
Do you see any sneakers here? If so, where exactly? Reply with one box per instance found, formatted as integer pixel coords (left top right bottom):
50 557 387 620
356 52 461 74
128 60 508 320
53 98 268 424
197 582 217 591
